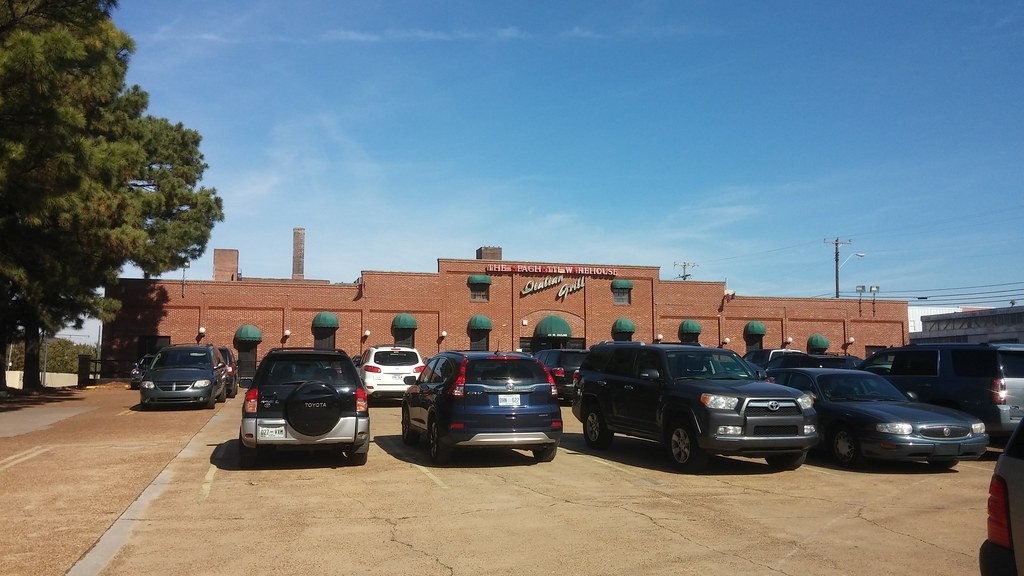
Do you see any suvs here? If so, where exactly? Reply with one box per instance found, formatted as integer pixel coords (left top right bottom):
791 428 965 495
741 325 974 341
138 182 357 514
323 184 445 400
751 352 865 379
739 348 805 366
240 347 370 464
356 345 428 405
571 340 819 471
848 340 1024 435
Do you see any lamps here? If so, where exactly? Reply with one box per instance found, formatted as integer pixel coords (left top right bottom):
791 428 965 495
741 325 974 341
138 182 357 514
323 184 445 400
722 290 734 302
656 334 664 342
442 331 446 336
365 330 370 336
784 337 792 345
721 338 731 344
846 337 855 346
284 330 292 337
199 328 207 335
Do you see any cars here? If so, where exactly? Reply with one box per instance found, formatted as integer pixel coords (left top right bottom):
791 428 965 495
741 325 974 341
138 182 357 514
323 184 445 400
130 354 156 390
535 349 590 406
764 369 990 470
139 343 228 410
401 350 563 467
217 345 240 398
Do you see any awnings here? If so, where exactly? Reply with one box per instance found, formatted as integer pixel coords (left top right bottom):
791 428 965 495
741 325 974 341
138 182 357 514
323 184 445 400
612 280 633 288
468 315 492 330
612 318 635 333
535 315 571 338
807 332 829 348
393 312 417 329
313 312 339 328
468 275 491 285
235 324 262 341
679 320 701 334
745 321 766 334
